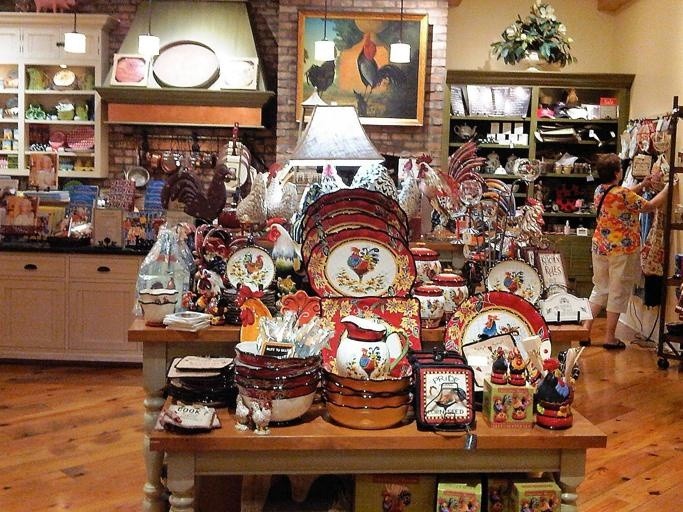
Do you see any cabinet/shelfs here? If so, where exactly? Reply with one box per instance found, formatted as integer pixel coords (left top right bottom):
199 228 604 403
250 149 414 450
0 254 145 365
437 68 638 318
652 97 682 374
0 11 121 180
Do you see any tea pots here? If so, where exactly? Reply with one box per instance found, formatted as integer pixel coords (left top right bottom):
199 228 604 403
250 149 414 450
77 69 94 90
24 65 51 92
452 120 477 142
75 100 89 120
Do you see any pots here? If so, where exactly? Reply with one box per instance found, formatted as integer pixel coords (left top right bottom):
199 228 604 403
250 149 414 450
127 121 251 192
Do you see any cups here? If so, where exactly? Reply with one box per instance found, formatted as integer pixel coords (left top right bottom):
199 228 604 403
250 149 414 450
540 151 595 176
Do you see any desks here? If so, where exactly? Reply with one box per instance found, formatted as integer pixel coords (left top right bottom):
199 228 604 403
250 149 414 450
124 312 590 512
147 400 609 510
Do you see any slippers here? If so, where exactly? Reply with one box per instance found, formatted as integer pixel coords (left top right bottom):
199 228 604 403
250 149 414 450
579 339 591 348
604 338 627 351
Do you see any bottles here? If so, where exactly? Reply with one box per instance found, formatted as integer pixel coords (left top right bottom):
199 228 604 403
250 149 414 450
0 155 8 168
562 219 571 236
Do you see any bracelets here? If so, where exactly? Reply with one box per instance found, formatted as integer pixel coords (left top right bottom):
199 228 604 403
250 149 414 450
639 182 642 190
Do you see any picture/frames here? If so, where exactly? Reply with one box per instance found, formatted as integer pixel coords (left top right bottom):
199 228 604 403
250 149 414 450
219 56 261 91
112 52 150 89
292 7 429 129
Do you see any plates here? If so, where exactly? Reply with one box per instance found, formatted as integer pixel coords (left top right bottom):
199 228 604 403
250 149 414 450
48 130 65 148
137 184 571 431
51 68 75 86
66 126 94 150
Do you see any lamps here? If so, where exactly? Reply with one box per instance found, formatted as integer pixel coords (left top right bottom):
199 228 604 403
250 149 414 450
134 0 161 59
61 1 90 57
387 0 412 64
285 105 386 172
313 0 336 61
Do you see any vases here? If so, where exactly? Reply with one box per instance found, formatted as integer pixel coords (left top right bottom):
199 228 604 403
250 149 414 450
519 45 553 73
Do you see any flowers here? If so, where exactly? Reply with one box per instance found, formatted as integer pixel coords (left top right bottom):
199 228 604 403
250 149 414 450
489 0 579 73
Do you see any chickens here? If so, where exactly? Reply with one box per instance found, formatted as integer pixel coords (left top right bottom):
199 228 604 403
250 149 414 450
195 262 224 296
236 395 254 425
251 399 272 433
236 162 421 237
353 90 368 117
264 218 304 275
357 33 408 101
235 284 274 342
160 164 232 224
416 139 546 262
306 60 336 98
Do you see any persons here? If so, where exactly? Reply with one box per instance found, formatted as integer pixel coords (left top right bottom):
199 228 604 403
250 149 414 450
578 152 679 350
15 199 34 227
37 156 54 188
